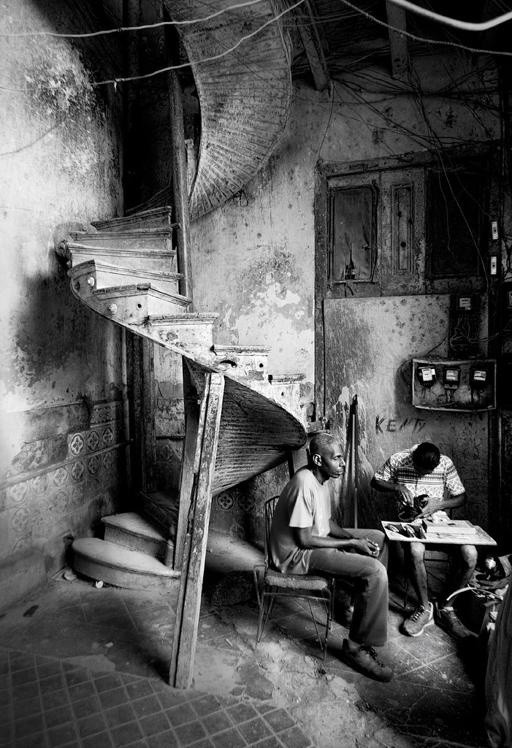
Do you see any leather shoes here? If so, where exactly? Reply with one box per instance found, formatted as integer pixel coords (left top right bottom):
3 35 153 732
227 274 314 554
398 494 430 522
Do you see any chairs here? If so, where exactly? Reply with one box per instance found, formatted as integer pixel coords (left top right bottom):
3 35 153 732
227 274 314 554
253 495 336 662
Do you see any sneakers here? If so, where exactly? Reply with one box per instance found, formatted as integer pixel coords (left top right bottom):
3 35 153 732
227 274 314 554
342 639 392 682
403 601 463 636
345 608 353 629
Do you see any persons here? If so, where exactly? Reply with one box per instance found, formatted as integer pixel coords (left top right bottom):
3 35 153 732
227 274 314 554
371 442 479 643
270 432 393 682
